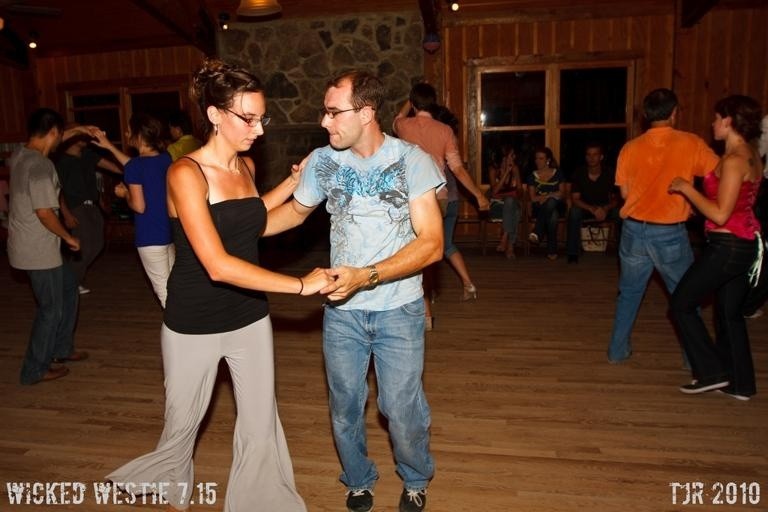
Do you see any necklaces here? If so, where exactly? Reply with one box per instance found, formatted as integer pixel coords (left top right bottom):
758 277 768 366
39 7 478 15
198 145 245 177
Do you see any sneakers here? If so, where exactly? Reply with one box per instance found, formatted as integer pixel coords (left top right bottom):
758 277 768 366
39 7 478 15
399 488 425 512
681 378 751 401
346 489 374 512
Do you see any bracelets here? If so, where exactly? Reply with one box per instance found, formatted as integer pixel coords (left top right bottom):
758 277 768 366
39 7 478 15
296 276 304 296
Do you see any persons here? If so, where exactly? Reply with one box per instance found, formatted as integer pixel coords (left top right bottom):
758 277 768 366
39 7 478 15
7 108 92 383
0 83 768 321
261 71 447 510
103 60 336 511
670 93 765 399
605 88 723 374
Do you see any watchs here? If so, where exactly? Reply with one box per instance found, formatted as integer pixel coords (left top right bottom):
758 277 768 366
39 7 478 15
364 265 379 290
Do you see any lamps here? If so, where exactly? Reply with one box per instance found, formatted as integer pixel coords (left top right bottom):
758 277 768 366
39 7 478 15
235 0 281 17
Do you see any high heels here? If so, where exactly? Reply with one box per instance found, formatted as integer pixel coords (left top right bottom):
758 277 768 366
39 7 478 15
463 284 477 300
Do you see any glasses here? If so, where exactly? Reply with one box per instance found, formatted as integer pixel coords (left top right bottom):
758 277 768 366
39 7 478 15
329 108 361 118
226 109 270 127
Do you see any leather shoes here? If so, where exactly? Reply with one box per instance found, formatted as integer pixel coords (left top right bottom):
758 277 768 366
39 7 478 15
52 351 86 362
38 368 69 381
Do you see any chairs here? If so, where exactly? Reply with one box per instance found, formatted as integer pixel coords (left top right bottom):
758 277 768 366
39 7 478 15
451 188 622 260
